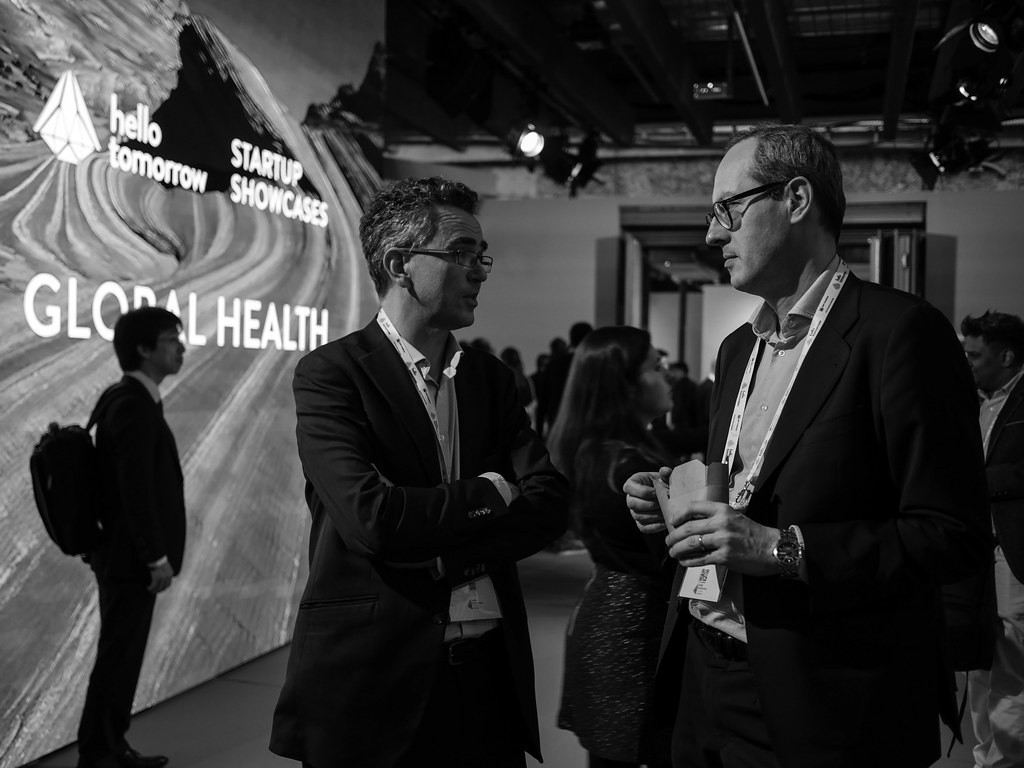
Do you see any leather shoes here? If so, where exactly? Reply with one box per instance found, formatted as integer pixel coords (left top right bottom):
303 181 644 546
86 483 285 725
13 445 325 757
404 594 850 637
77 748 168 768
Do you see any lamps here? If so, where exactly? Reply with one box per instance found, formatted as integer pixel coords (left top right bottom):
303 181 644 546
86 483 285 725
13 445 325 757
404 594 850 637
503 118 545 158
970 1 1024 52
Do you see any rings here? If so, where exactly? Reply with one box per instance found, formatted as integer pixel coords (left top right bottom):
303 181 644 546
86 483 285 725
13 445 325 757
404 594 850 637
697 535 706 551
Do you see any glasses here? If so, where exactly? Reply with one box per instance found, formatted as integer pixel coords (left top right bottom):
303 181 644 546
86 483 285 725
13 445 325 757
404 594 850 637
705 180 787 230
399 248 494 273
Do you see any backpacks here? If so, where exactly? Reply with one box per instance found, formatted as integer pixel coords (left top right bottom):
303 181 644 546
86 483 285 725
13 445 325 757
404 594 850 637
30 385 147 556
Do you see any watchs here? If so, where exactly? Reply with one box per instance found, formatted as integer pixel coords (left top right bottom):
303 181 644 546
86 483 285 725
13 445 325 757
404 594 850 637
770 526 802 578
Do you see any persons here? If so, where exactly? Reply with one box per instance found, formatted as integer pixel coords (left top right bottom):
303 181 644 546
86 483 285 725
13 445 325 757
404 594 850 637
265 175 564 768
77 307 187 768
454 124 1024 768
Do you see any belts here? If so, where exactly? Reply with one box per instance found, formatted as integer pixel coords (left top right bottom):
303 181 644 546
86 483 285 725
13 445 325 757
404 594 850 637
993 533 1000 546
437 639 475 665
696 622 748 660
